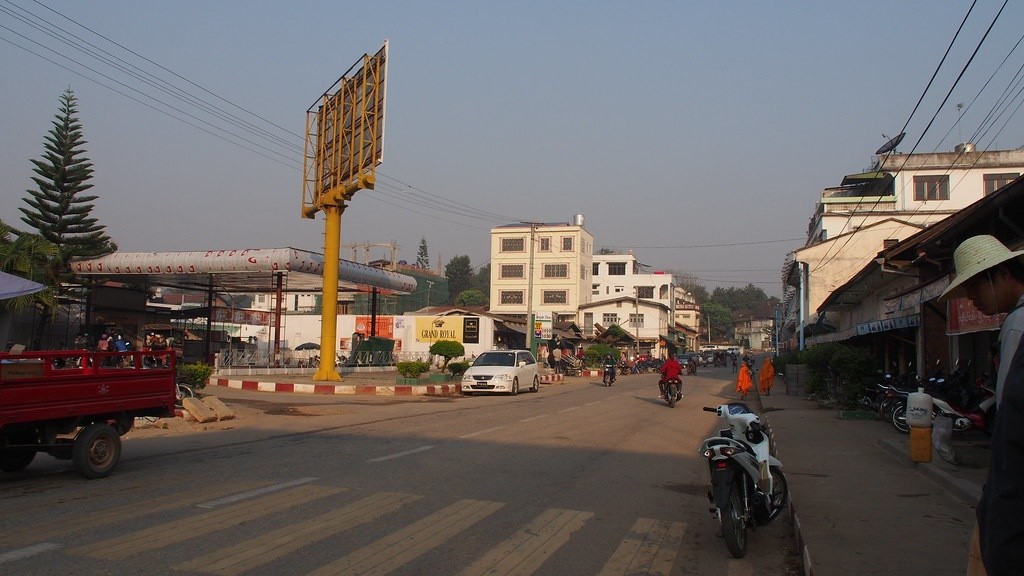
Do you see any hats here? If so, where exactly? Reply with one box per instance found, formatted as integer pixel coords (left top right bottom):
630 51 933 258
579 347 583 349
118 335 121 339
102 334 107 338
937 235 1024 302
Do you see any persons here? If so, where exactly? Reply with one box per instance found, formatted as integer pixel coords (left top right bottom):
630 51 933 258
759 357 775 396
285 355 320 368
601 346 777 384
736 360 752 400
60 327 184 370
938 234 1024 576
657 352 685 398
553 346 584 375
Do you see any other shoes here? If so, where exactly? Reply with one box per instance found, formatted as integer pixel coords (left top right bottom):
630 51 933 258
657 394 685 398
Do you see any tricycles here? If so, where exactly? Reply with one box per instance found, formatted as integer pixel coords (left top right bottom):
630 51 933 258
713 349 727 369
0 349 177 481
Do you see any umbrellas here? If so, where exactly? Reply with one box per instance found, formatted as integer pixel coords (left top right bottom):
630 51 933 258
296 343 320 358
0 271 47 299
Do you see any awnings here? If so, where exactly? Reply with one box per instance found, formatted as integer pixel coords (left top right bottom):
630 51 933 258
804 314 921 345
502 322 527 334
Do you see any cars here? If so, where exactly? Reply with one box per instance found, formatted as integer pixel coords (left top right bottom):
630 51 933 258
698 345 741 365
460 350 541 396
676 354 689 370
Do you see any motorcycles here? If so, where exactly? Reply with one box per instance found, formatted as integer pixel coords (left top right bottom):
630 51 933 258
876 359 998 438
697 401 788 559
602 363 617 388
659 371 683 408
684 360 698 375
